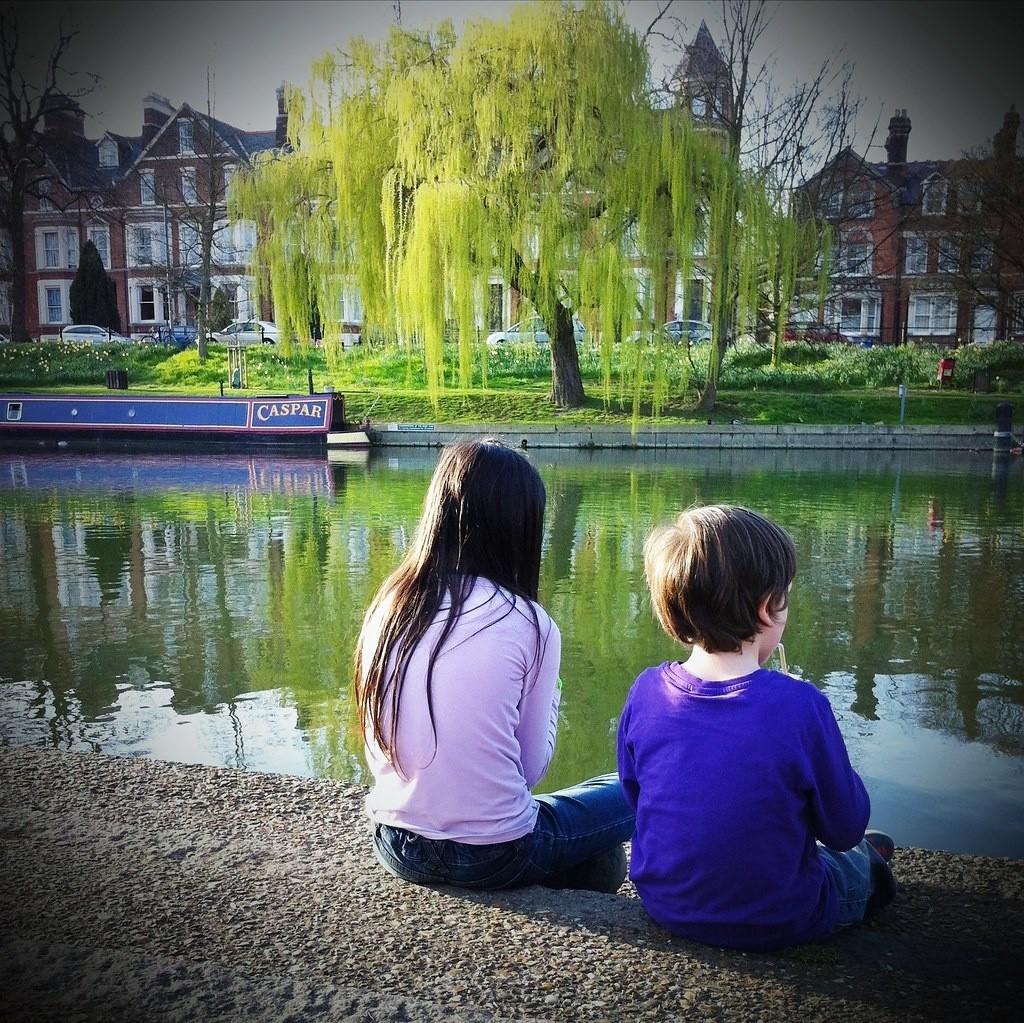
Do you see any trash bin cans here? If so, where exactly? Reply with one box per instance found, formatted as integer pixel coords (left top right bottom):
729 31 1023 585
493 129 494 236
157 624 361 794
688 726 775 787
105 370 128 389
936 359 955 380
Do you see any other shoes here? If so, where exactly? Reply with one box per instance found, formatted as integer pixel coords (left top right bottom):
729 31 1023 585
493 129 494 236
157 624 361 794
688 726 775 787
863 830 894 862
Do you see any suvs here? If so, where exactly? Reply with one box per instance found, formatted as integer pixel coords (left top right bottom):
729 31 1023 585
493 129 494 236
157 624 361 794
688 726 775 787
338 321 362 352
486 316 589 344
155 326 198 349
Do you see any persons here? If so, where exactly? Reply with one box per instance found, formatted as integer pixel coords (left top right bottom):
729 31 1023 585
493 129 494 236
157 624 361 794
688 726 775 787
355 440 636 897
616 504 896 961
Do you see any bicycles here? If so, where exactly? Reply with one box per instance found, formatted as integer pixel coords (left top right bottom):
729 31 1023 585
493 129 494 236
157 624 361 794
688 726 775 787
141 328 178 345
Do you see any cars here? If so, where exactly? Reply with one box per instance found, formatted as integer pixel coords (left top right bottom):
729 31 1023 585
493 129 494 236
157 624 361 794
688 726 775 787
57 324 135 344
783 321 852 348
625 319 734 348
205 320 300 346
